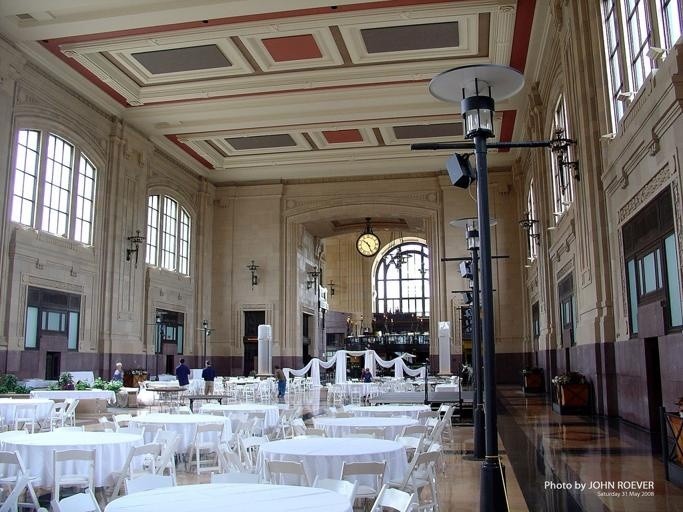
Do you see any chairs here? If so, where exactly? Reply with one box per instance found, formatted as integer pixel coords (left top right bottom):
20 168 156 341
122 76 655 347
0 375 463 512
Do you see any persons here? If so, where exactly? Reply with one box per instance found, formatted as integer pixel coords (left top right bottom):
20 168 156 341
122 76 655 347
201 361 218 403
112 362 124 383
176 357 191 407
274 366 286 398
364 368 373 403
361 368 365 379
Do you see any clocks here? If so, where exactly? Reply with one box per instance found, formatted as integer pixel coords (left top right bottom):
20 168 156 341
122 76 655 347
356 217 380 257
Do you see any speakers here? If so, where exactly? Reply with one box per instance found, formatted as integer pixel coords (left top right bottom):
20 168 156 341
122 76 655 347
445 153 476 189
459 260 473 278
463 293 472 304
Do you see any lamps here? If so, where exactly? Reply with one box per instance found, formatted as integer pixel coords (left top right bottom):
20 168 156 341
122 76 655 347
327 279 335 299
307 266 321 295
126 228 145 264
245 260 260 292
